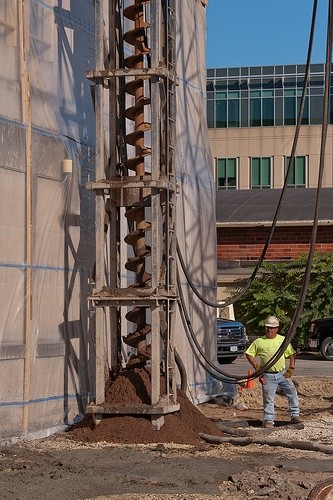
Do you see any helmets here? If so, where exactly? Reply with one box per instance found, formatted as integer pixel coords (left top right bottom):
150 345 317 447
264 315 279 328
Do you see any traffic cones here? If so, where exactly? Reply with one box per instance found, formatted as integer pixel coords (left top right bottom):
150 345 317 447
243 370 255 389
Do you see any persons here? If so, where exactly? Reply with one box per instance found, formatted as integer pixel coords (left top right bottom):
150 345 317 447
245 316 304 428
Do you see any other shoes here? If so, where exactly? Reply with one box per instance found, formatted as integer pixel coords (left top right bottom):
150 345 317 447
290 416 305 429
262 422 275 429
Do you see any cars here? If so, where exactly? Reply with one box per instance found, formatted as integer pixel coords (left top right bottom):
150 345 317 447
312 317 333 361
216 318 247 364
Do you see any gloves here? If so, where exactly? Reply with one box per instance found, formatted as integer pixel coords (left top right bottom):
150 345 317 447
282 366 295 378
252 364 264 378
